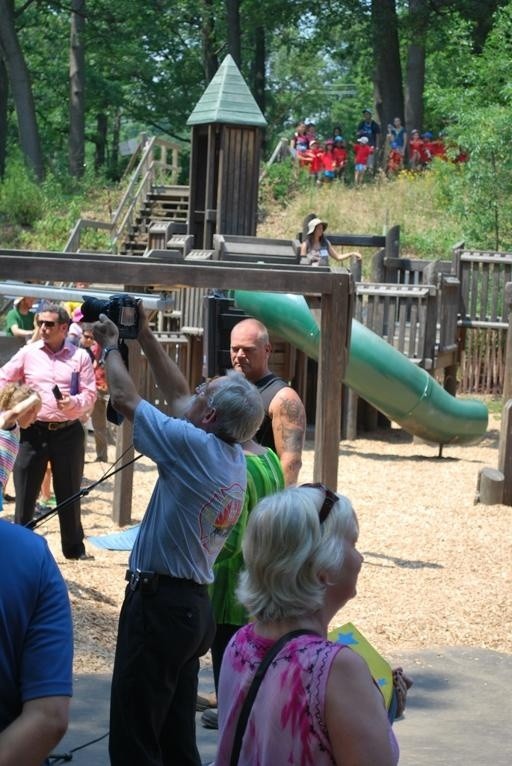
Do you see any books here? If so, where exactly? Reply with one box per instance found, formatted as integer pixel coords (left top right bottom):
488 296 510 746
324 621 397 717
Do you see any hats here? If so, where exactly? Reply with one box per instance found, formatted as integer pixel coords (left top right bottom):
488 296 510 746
303 108 443 150
71 307 84 323
306 217 328 236
13 296 24 306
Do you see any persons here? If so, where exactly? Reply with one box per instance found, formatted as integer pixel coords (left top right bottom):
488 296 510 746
299 216 363 267
193 431 289 731
81 295 266 764
0 279 110 509
213 479 417 765
0 304 99 563
287 106 469 190
0 520 76 766
229 315 309 489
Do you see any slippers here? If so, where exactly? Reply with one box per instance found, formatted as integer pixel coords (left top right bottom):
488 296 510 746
40 495 57 506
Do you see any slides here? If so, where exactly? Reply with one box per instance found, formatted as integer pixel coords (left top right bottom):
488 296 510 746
234 288 487 444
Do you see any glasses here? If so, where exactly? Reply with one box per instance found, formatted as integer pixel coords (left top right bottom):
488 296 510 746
36 320 60 328
299 481 340 527
82 334 95 340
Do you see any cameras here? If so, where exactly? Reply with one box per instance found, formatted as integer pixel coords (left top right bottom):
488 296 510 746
80 294 138 340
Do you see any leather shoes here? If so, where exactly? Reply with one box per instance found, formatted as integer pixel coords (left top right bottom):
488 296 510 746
78 553 95 561
195 689 219 712
200 707 219 730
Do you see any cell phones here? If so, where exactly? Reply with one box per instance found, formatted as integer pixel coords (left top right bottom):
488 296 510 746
52 384 62 400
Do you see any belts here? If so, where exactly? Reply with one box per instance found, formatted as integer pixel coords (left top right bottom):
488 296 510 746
125 570 192 583
35 419 78 432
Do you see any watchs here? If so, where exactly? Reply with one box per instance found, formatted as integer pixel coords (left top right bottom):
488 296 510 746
101 342 120 362
